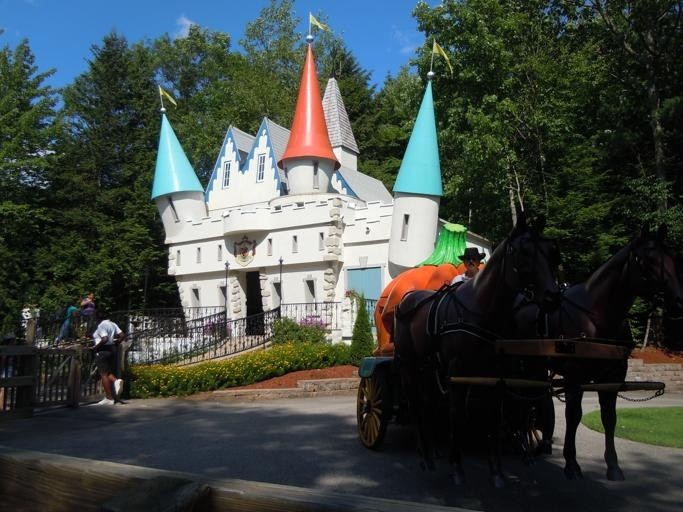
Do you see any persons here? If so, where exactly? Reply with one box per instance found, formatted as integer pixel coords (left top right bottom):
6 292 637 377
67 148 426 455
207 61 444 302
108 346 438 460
78 290 98 340
450 246 487 287
82 308 128 407
57 304 80 341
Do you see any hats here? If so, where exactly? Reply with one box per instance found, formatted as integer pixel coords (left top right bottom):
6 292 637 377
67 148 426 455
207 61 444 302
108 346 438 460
458 247 486 261
67 306 77 318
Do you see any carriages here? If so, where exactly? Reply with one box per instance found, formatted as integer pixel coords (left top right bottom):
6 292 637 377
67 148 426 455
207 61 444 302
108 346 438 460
357 209 683 490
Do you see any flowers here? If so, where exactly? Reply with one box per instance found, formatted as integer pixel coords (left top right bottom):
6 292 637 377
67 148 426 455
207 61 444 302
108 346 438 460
299 316 325 329
203 317 231 337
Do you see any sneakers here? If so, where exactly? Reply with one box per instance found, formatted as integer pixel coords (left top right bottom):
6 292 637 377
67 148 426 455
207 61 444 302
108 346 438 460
98 396 115 406
114 378 123 396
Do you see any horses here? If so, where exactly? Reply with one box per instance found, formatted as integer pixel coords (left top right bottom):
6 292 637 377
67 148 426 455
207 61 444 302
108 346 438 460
391 209 566 489
512 218 683 482
